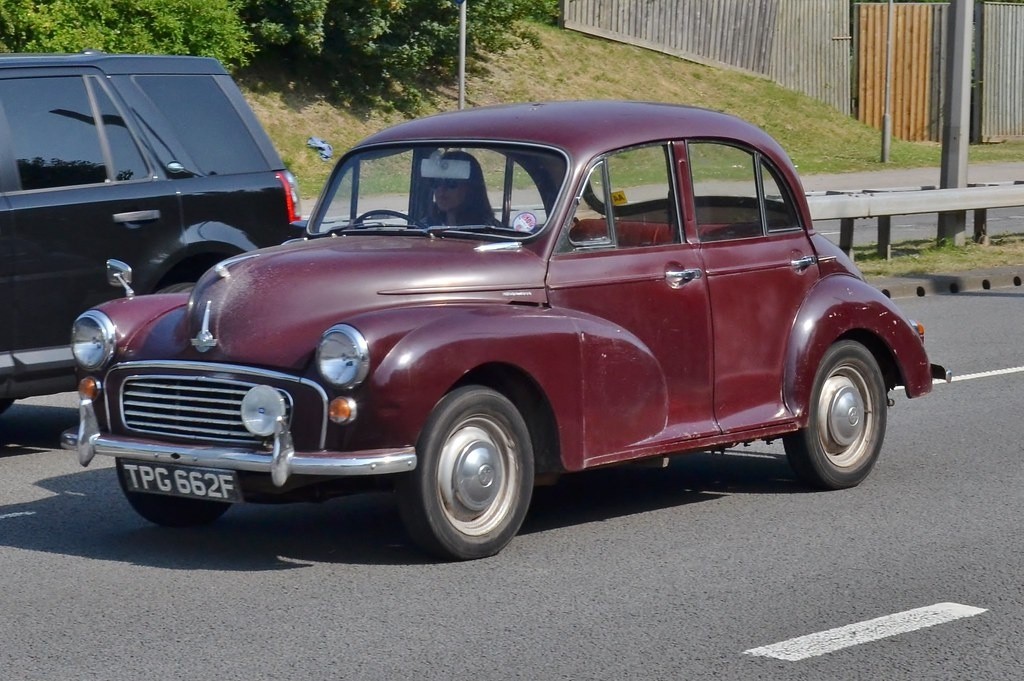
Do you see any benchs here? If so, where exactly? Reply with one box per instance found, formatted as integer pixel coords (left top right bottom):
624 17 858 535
570 219 769 248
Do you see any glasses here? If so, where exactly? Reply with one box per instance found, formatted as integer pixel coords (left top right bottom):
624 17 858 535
429 178 472 189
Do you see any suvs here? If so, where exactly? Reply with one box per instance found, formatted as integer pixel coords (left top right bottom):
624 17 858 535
0 52 301 422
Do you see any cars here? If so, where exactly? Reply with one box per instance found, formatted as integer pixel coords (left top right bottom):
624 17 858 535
59 100 952 562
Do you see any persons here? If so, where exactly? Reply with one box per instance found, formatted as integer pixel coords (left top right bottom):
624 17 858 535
413 150 504 230
